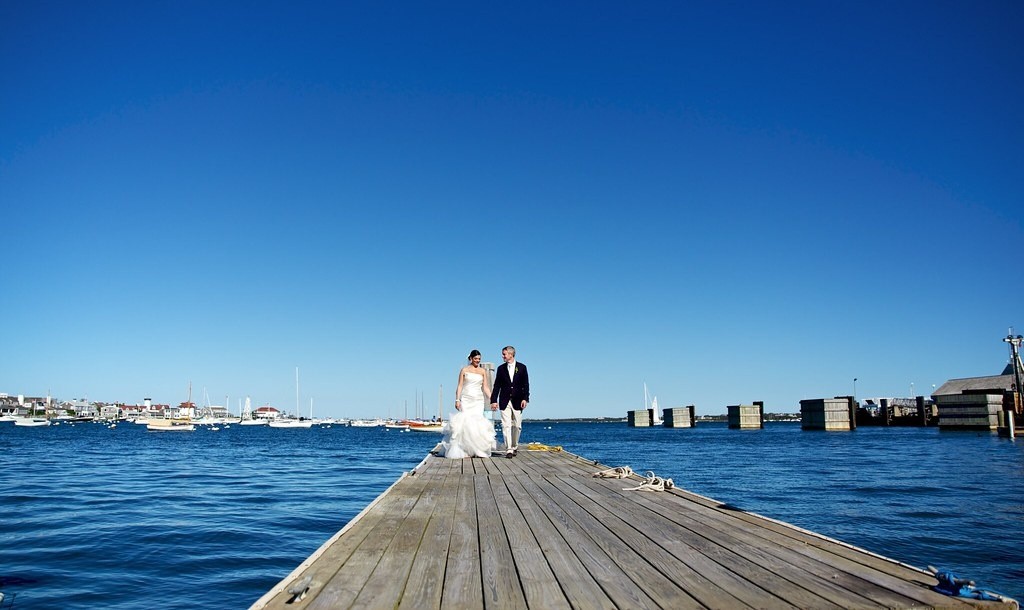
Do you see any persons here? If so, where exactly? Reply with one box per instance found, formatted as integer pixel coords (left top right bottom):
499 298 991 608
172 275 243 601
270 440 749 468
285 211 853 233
490 346 530 458
455 346 498 458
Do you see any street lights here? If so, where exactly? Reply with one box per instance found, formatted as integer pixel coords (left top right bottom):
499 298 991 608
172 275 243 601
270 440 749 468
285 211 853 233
853 378 857 401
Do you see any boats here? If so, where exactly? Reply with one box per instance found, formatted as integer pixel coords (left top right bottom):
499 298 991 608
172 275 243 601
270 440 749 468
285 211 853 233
0 366 449 433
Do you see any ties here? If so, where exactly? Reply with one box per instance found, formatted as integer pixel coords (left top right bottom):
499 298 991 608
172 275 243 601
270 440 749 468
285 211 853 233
509 365 514 383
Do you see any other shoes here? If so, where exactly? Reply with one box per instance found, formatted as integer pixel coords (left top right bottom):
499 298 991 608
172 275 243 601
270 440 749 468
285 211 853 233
506 453 512 458
513 450 518 456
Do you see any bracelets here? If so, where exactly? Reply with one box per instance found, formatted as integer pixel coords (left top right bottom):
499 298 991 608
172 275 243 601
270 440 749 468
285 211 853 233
455 400 460 401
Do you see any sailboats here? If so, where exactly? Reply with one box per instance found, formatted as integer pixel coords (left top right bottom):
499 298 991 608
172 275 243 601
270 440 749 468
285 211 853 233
651 396 664 426
